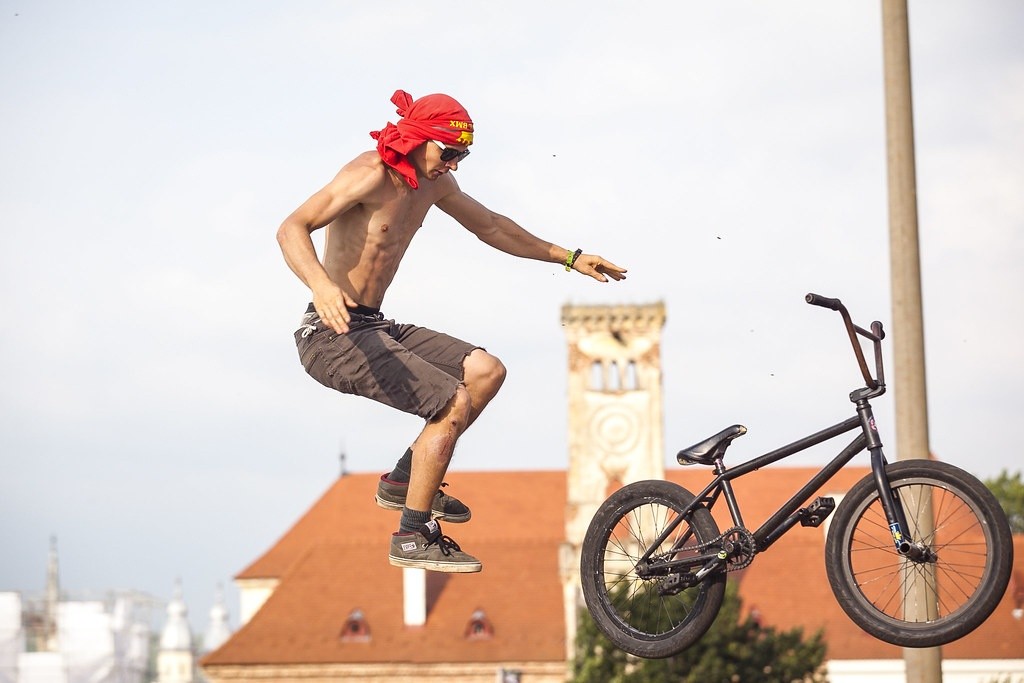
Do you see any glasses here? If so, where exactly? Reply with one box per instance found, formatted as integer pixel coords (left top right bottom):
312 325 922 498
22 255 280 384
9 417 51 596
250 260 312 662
432 139 471 163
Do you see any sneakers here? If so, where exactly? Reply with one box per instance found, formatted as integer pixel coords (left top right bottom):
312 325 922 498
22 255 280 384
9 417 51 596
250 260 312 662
375 473 471 524
390 519 483 573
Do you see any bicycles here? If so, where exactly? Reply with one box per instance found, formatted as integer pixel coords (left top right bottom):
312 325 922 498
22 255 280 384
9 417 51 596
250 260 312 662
580 293 1014 659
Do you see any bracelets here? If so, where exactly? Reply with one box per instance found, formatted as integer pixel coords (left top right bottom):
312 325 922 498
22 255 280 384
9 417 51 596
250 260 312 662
565 249 574 272
571 248 583 269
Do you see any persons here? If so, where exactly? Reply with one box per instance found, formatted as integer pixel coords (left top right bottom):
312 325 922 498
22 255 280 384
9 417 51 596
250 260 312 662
275 88 626 572
